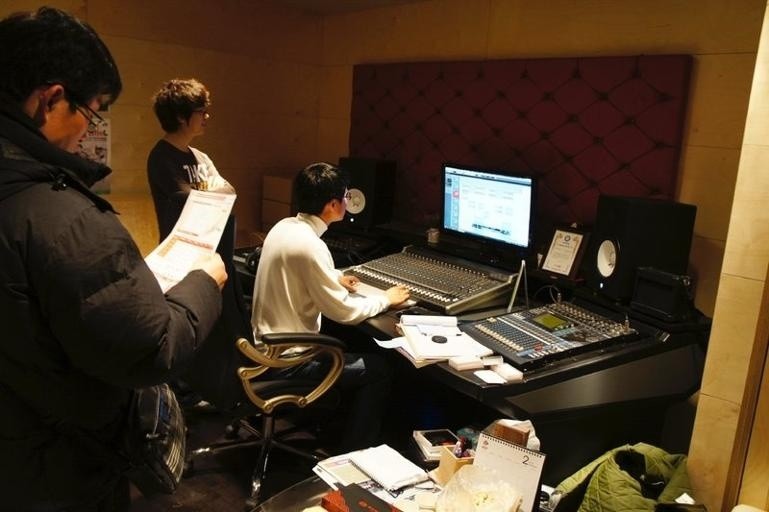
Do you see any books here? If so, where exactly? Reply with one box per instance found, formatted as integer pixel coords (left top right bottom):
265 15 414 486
413 429 466 461
395 315 491 370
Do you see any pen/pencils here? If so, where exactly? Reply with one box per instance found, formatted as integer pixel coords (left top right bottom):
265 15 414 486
453 436 470 457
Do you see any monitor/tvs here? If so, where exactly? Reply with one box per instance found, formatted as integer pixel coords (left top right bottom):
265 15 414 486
441 161 537 267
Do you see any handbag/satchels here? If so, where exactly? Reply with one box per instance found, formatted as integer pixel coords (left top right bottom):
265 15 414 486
122 383 186 501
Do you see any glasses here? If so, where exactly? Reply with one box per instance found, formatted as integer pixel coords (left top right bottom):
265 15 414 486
44 77 103 132
329 192 351 201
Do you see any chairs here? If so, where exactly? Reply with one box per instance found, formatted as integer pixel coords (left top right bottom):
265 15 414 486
194 240 350 509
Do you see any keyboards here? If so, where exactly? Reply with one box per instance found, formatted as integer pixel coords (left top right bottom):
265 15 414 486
351 282 386 298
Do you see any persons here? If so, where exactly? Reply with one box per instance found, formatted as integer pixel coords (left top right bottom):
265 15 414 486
1 6 227 512
148 78 238 417
250 161 409 443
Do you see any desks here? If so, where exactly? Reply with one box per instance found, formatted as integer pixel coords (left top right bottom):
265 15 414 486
234 227 708 442
244 448 556 512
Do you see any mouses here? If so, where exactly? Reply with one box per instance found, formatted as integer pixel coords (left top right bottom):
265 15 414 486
396 309 420 318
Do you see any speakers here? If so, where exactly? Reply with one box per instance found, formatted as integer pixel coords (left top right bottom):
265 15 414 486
338 157 397 226
580 193 697 304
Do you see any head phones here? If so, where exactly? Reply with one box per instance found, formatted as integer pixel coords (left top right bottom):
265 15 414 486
246 247 261 274
